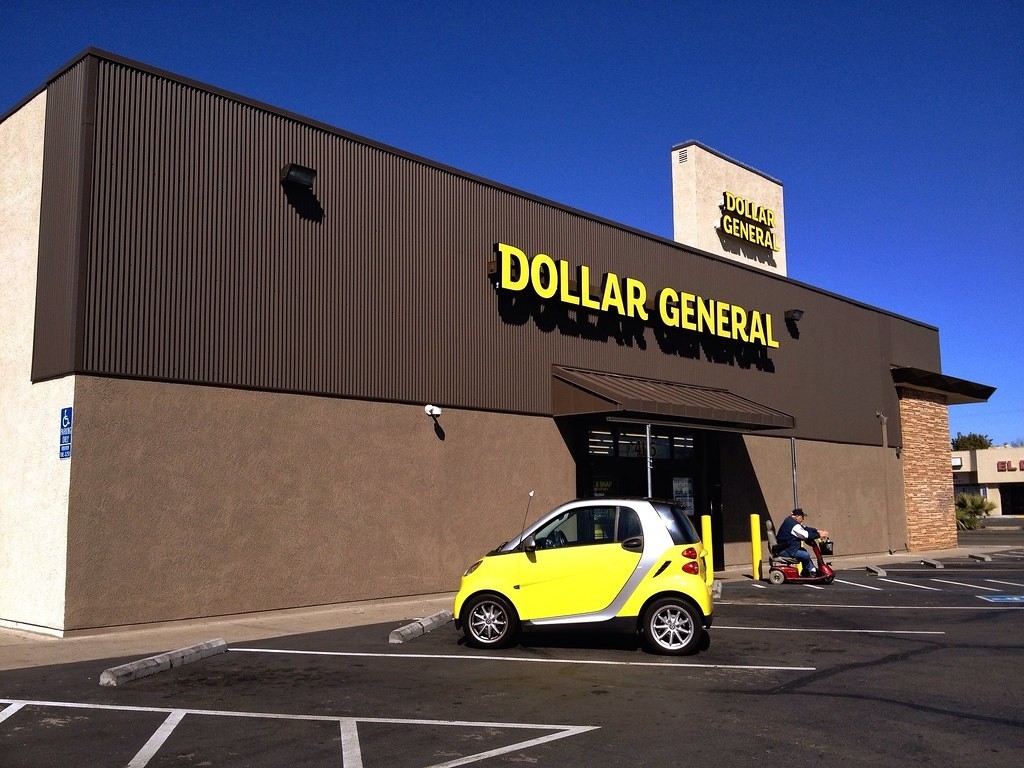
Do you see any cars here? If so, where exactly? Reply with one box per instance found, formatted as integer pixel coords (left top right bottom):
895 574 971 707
454 498 714 655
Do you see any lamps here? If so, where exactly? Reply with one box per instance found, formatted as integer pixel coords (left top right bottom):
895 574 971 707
424 404 442 415
280 162 317 188
784 308 804 320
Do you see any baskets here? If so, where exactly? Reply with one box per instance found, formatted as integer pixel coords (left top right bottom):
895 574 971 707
820 542 833 555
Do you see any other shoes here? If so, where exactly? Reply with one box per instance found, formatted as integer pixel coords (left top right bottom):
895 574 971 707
803 568 818 577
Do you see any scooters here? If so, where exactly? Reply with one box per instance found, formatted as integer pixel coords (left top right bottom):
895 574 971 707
765 520 836 585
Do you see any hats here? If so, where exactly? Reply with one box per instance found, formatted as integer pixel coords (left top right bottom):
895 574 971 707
791 509 807 516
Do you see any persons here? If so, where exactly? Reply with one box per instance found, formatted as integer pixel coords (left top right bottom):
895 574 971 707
776 508 831 578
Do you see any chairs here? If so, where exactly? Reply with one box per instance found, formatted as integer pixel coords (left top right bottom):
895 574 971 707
765 518 799 564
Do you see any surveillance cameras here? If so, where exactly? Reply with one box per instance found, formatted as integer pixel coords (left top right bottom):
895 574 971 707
432 409 442 417
